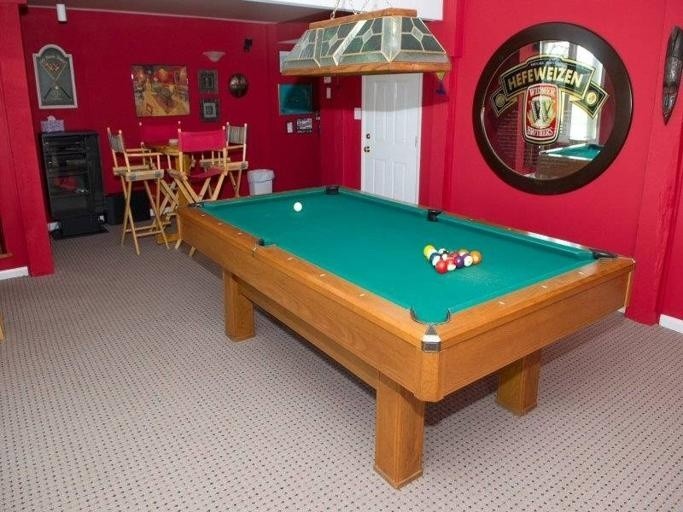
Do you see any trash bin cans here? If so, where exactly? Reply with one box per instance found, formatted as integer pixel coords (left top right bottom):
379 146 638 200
247 169 275 196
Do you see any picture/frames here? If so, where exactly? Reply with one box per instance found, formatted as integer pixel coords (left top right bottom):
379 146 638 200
197 69 219 94
199 98 221 123
277 83 314 117
32 43 78 110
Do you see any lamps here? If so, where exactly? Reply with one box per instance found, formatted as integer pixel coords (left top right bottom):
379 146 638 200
202 51 226 63
56 3 68 23
281 1 452 77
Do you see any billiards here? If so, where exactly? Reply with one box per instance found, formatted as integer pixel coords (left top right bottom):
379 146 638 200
424 245 482 274
293 202 303 212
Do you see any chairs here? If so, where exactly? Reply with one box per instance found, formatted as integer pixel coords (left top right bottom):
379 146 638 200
166 125 228 257
199 121 249 200
106 127 170 255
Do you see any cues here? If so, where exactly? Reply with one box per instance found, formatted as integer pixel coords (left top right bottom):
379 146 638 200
44 61 70 100
39 63 72 100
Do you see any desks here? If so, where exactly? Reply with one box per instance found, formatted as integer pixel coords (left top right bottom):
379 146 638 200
535 142 605 181
141 144 195 245
176 183 638 491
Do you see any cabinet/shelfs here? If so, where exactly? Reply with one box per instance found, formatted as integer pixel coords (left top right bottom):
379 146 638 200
37 129 106 241
